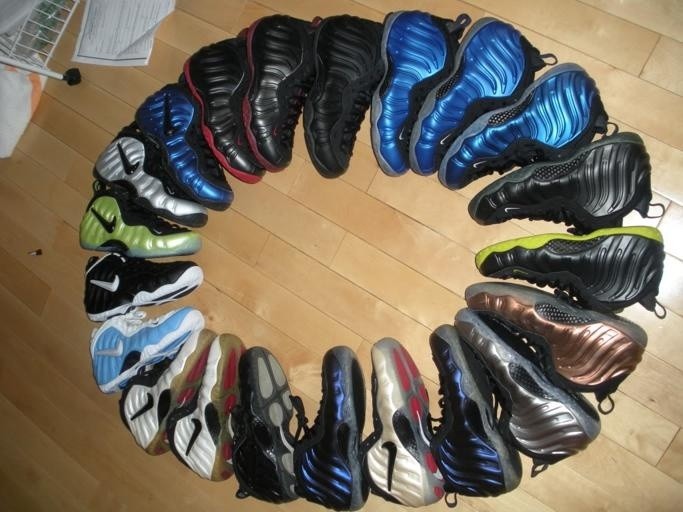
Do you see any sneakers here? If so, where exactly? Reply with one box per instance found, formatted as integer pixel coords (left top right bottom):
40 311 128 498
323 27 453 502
84 227 665 511
79 9 665 257
